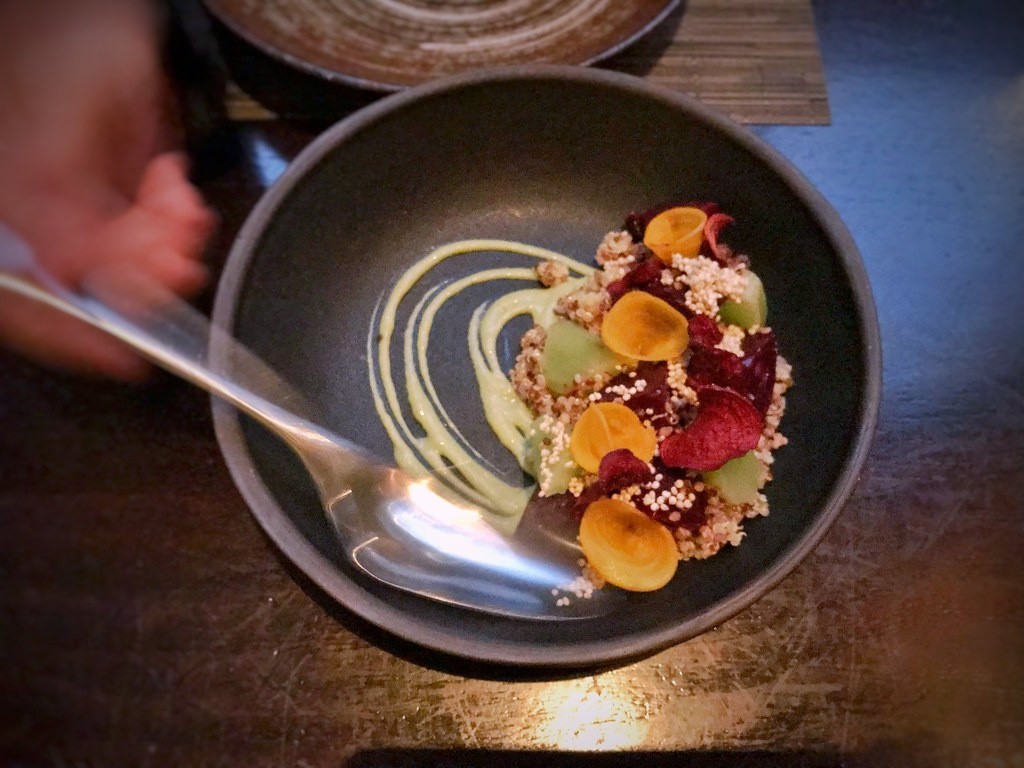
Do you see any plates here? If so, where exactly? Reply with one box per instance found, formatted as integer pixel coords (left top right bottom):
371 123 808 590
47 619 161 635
192 0 684 110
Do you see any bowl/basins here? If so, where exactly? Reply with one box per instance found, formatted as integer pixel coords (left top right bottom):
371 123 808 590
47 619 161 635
210 63 883 670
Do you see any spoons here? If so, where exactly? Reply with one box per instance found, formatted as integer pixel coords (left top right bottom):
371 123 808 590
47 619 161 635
0 194 630 621
